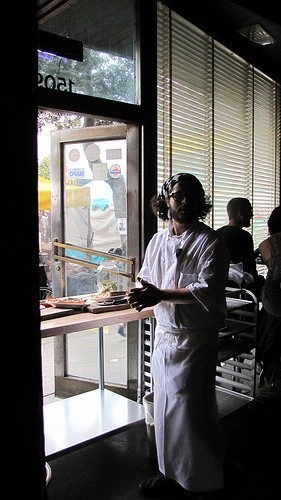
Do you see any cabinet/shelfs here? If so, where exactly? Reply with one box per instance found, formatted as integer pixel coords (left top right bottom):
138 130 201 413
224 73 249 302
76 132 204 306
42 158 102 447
39 294 155 462
138 287 259 422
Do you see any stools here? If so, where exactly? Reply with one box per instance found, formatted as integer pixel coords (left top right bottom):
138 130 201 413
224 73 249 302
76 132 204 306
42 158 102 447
258 311 281 387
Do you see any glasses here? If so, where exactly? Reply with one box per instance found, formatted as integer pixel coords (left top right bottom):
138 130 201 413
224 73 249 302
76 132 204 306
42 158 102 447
169 193 201 201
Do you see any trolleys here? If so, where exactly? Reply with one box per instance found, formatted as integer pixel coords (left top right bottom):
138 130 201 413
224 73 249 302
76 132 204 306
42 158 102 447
140 286 258 426
40 304 152 485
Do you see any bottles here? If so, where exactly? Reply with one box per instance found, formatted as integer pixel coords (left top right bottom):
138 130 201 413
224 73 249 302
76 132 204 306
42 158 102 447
97 260 119 298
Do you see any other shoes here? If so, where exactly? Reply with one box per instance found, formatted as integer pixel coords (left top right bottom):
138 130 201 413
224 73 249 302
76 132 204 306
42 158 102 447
138 473 175 489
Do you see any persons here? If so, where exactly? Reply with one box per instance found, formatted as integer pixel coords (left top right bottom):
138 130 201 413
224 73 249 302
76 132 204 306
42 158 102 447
126 173 230 500
39 209 50 230
215 198 259 291
256 206 281 390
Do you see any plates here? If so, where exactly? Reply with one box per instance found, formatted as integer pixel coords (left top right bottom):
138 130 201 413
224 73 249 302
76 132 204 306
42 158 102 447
45 301 89 308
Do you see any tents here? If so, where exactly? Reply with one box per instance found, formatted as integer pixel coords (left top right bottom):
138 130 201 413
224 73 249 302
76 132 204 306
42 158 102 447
38 176 93 264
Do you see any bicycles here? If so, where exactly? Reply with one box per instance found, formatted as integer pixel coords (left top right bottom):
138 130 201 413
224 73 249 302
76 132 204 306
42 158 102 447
39 287 53 300
